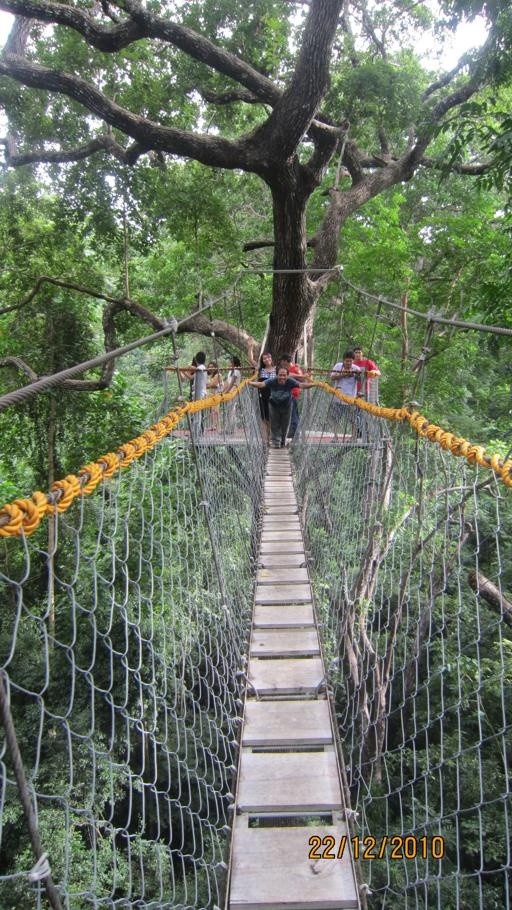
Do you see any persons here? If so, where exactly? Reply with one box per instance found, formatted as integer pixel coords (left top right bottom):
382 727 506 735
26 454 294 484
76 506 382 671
179 337 317 449
352 347 381 439
329 351 362 444
